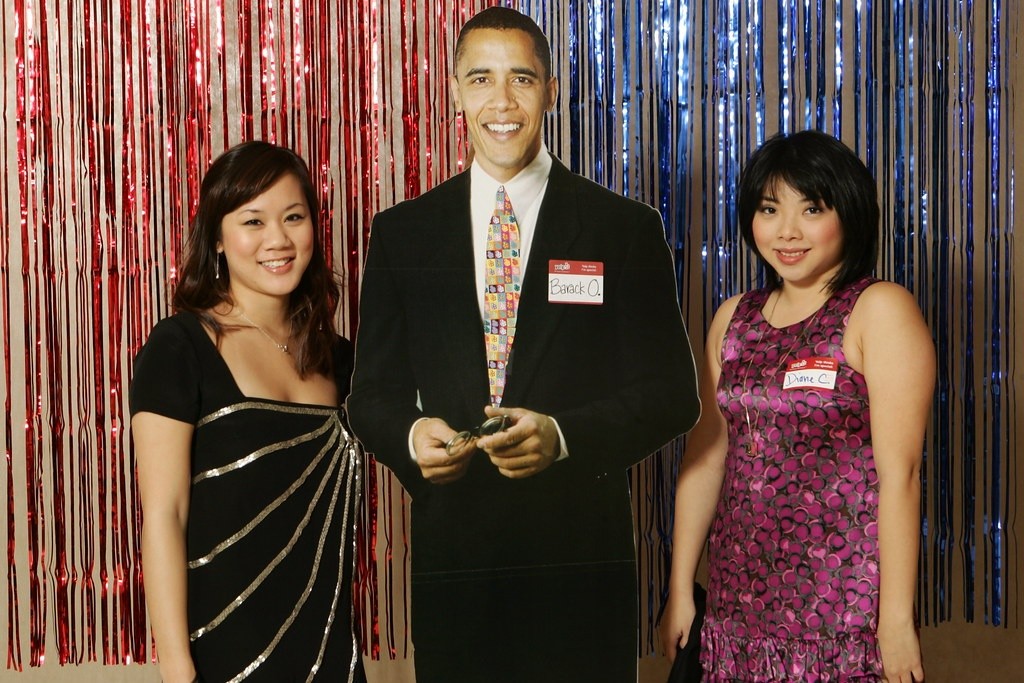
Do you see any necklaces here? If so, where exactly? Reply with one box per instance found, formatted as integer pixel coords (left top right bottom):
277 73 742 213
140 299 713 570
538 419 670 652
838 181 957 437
234 306 293 354
741 289 820 456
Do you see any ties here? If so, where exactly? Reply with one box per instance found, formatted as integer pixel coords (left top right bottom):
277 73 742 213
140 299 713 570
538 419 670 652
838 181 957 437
485 185 523 409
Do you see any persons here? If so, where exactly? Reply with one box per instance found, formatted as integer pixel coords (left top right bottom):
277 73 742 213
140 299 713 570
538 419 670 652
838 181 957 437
346 7 702 683
130 141 368 682
658 131 937 683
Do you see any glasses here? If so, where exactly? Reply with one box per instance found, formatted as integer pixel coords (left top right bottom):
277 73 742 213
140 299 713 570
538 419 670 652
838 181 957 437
446 415 510 455
